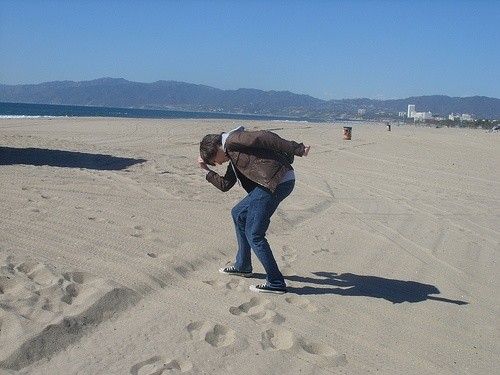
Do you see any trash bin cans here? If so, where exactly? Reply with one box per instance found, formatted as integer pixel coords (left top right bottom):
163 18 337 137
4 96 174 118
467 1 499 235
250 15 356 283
342 126 353 140
386 124 391 131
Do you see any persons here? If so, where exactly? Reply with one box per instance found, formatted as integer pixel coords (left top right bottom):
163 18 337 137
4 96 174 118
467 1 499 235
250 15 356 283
197 125 312 295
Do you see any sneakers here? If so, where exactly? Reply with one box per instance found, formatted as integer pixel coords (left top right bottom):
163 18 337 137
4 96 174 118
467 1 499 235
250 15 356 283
249 284 286 294
220 266 253 277
